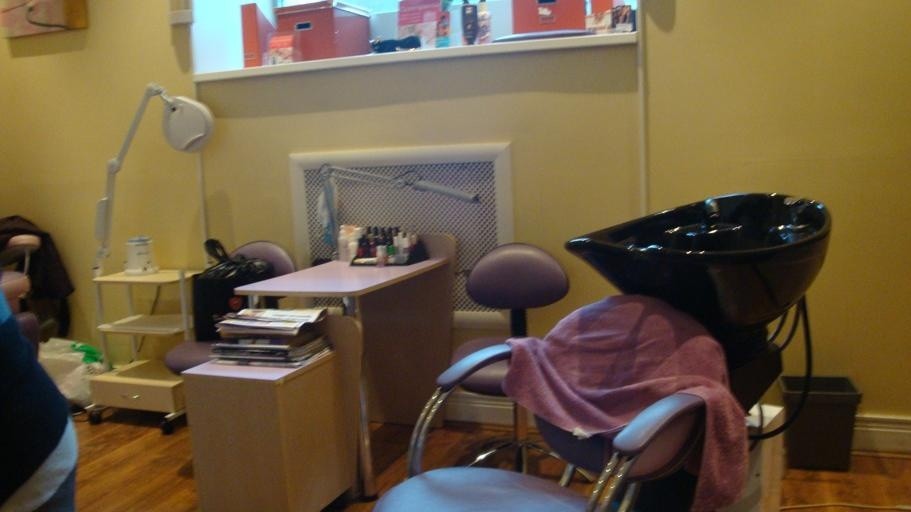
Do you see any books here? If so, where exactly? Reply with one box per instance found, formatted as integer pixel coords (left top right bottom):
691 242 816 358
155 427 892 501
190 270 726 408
205 307 333 368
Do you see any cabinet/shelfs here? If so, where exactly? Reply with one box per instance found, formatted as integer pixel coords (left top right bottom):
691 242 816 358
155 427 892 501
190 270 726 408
182 350 359 511
88 268 203 434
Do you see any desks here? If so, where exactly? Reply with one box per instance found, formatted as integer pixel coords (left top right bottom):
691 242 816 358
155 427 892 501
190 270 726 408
234 233 459 502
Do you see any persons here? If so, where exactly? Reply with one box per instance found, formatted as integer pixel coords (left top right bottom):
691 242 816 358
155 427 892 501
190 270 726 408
1 288 77 512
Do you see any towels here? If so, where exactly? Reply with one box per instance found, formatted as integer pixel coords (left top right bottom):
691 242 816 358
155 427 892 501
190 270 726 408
506 292 749 511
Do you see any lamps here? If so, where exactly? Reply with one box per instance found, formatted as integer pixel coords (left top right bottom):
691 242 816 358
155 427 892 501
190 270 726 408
93 82 215 369
319 165 478 258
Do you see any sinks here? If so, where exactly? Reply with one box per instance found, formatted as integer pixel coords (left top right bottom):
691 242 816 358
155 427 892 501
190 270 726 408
564 192 833 326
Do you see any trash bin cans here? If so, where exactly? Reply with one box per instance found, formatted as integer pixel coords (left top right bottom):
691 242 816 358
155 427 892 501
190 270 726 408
775 372 863 471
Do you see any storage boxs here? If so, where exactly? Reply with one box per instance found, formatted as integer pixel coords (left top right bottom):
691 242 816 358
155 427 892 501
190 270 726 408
273 0 370 58
513 0 586 30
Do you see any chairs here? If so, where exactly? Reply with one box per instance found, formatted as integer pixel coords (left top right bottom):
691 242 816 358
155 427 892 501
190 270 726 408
372 296 722 512
164 240 295 376
450 243 593 485
